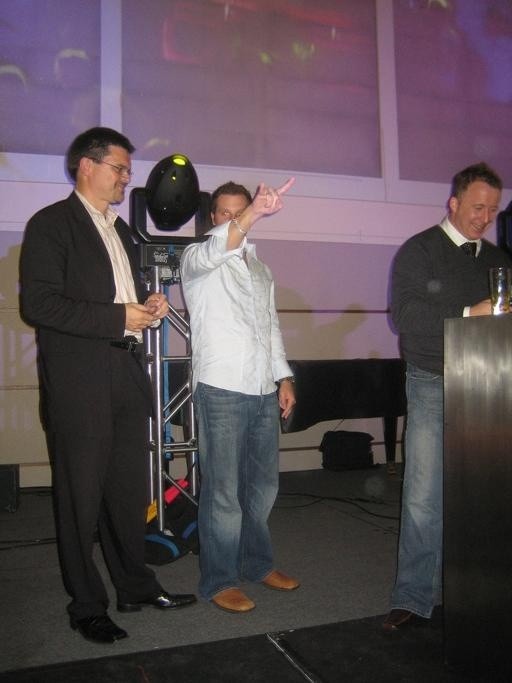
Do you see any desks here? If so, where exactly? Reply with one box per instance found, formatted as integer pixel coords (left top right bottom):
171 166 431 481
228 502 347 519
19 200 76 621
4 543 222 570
144 358 410 464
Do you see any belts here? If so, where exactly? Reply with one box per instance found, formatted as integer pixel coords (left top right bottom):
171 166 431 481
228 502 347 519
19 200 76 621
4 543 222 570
109 337 139 353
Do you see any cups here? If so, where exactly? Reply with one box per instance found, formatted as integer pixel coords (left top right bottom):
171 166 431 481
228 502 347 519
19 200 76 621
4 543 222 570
488 267 511 316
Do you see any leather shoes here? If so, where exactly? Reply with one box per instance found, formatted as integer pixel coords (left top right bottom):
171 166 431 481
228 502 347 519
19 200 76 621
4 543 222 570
380 606 418 633
70 611 130 644
211 585 257 613
257 566 302 590
116 588 199 612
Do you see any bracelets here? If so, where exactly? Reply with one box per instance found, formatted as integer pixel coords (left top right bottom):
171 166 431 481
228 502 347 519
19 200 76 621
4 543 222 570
279 376 296 383
231 218 248 234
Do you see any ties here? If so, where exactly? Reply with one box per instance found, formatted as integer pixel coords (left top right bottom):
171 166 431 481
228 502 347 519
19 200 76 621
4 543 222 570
460 241 477 259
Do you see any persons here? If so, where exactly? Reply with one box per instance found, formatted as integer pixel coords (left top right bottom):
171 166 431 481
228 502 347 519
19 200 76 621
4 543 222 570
18 126 197 644
178 176 301 612
381 162 512 633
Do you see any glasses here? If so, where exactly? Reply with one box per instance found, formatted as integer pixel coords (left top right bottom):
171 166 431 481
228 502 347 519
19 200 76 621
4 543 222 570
88 155 131 174
